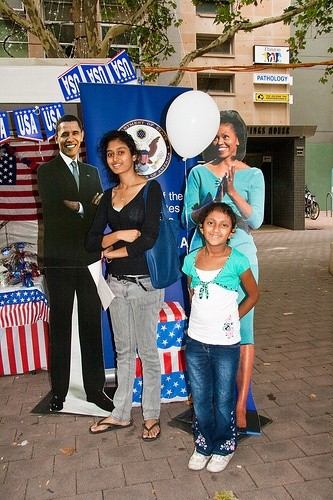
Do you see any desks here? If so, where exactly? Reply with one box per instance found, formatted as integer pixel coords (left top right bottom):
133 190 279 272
0 274 50 377
131 301 192 407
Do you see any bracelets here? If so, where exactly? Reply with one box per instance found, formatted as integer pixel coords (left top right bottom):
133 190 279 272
106 252 112 263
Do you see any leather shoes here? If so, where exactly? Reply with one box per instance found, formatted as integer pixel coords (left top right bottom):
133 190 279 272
49 395 65 411
87 391 115 412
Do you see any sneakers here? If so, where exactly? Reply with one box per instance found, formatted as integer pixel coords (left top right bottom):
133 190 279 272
187 447 213 471
206 452 235 473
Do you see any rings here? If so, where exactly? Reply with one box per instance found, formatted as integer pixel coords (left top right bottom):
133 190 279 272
230 179 232 181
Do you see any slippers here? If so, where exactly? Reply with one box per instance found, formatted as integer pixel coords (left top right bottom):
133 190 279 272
142 419 162 442
89 417 133 433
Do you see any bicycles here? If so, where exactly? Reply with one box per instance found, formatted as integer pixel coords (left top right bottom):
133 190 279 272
305 185 320 220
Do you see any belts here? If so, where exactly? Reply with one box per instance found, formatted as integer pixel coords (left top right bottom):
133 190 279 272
112 275 151 292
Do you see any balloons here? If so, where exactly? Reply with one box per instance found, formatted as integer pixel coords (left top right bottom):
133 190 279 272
166 90 220 162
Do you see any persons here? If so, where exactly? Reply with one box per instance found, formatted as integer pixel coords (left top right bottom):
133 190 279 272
181 202 261 473
180 111 266 435
85 130 165 442
36 114 116 413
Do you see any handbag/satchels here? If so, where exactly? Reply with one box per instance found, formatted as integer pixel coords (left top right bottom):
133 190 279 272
142 219 184 289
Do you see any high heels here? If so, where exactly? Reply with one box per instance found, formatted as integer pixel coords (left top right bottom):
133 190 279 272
236 413 248 432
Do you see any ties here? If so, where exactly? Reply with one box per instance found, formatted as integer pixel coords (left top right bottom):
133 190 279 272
70 161 80 192
